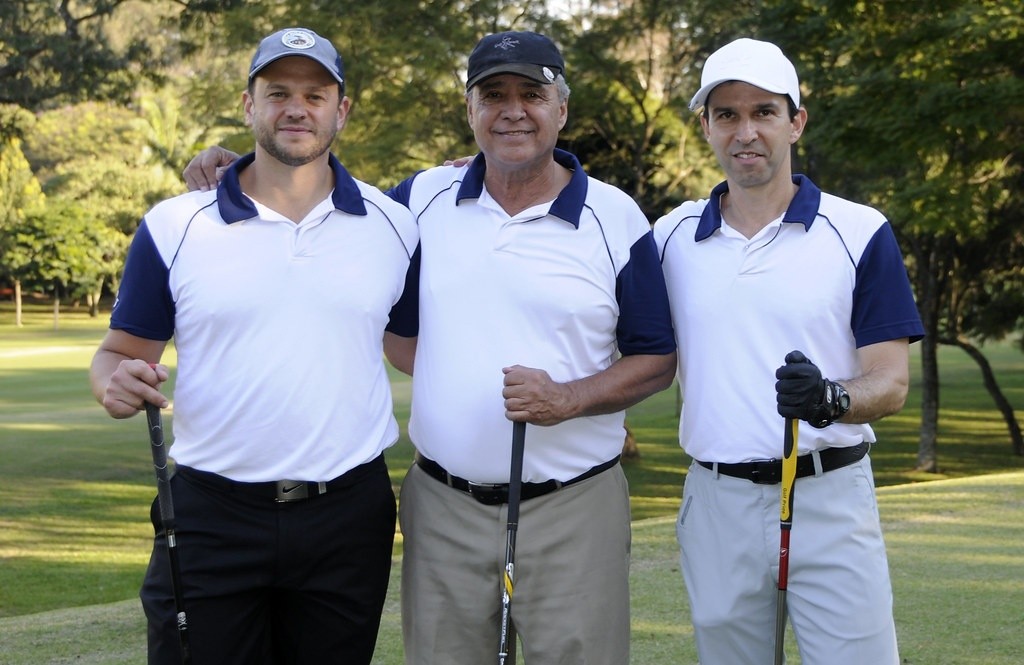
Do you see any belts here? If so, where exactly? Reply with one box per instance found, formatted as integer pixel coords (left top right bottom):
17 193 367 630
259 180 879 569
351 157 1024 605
696 439 871 486
175 451 381 503
414 450 619 506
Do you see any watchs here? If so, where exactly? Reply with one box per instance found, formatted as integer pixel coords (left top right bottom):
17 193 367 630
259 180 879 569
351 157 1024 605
831 381 850 421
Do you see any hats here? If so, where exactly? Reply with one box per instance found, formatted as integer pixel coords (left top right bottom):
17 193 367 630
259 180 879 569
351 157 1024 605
466 30 564 84
246 28 347 88
689 38 800 111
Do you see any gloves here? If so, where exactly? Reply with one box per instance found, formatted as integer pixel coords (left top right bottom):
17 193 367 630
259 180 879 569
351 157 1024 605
772 351 835 429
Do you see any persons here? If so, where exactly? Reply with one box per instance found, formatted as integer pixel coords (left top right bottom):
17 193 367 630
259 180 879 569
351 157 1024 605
91 27 421 665
184 31 676 665
654 37 925 663
